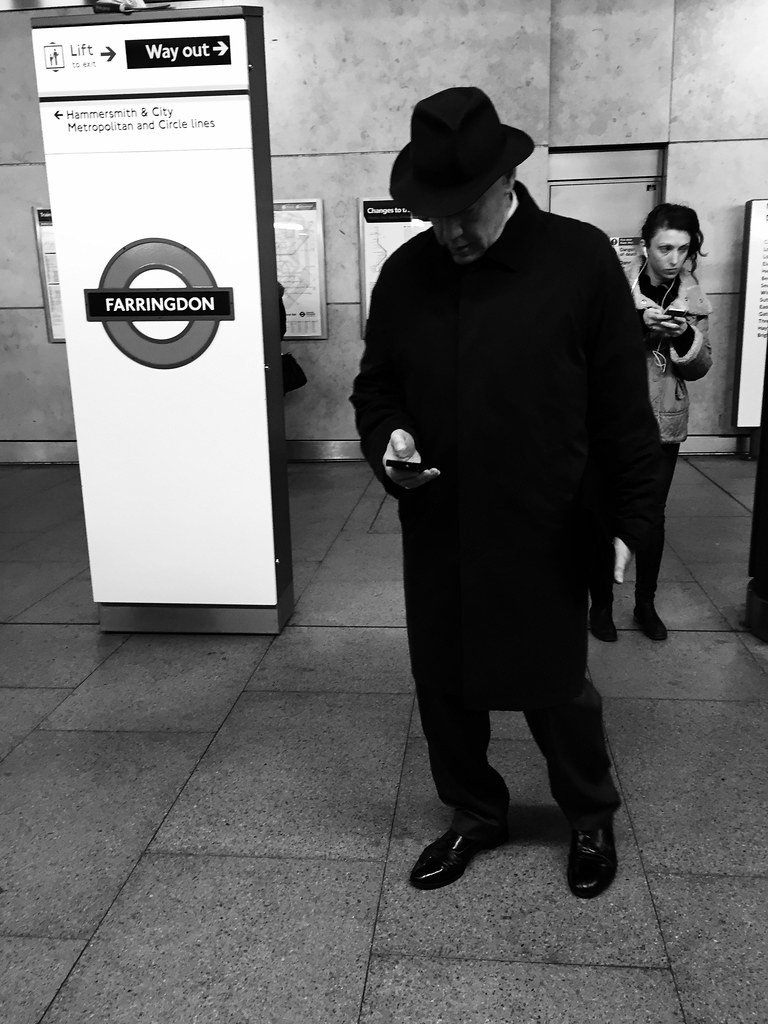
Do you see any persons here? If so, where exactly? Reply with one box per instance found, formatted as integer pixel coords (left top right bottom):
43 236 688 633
348 86 660 899
277 280 287 340
589 201 715 643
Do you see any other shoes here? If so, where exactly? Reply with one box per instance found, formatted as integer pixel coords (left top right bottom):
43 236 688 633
634 601 667 640
589 602 617 641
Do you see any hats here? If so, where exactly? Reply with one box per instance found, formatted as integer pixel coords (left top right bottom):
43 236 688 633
390 87 535 217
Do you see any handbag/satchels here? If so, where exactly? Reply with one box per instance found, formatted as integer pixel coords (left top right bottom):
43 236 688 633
282 352 307 394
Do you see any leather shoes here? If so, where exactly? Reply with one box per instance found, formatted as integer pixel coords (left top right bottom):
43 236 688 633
567 815 618 898
410 828 508 890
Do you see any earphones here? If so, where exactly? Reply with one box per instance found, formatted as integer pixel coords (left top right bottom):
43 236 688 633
643 246 648 258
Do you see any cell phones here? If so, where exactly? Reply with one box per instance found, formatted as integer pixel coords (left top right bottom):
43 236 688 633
385 459 436 471
661 308 688 321
